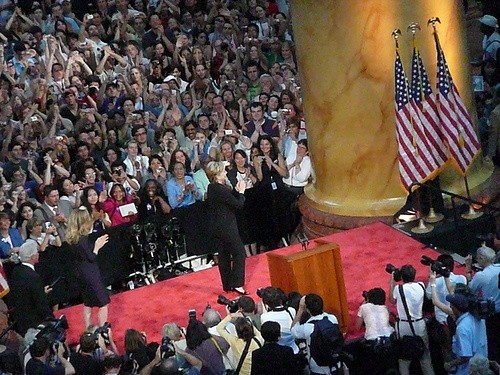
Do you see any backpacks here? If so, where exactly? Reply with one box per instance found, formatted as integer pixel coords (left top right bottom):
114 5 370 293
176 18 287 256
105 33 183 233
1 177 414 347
308 316 346 367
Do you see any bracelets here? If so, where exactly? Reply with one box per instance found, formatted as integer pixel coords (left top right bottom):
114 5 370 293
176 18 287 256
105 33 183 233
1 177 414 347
467 271 472 273
53 163 55 167
431 284 436 287
54 233 59 236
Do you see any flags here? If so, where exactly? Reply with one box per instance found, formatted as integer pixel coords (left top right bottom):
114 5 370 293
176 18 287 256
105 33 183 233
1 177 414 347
408 31 448 183
432 29 482 177
389 38 441 194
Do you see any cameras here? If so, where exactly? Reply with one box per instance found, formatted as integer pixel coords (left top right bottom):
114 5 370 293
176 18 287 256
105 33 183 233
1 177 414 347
386 263 402 282
160 336 172 352
421 255 450 278
87 14 94 20
361 290 369 303
217 294 239 313
95 321 111 345
189 309 196 322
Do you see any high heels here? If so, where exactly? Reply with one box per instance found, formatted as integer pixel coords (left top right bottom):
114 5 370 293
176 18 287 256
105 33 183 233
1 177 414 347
226 286 250 295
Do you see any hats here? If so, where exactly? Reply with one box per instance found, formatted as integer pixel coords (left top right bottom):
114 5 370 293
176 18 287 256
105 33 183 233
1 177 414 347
446 293 469 312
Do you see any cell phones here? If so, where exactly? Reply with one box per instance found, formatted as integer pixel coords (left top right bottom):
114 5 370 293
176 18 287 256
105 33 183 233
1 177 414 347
46 222 51 229
116 169 121 176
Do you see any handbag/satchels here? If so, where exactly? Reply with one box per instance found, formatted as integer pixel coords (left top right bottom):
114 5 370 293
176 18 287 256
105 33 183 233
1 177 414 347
395 335 426 361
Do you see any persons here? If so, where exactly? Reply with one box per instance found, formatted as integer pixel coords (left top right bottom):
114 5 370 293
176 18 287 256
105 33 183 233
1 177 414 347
205 161 250 297
9 238 55 334
0 0 500 375
63 207 117 347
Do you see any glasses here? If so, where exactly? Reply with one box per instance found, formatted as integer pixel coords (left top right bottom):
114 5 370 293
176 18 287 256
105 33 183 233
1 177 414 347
85 171 96 176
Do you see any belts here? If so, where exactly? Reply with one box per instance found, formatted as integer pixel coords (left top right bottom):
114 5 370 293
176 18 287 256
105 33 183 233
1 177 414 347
401 317 423 322
